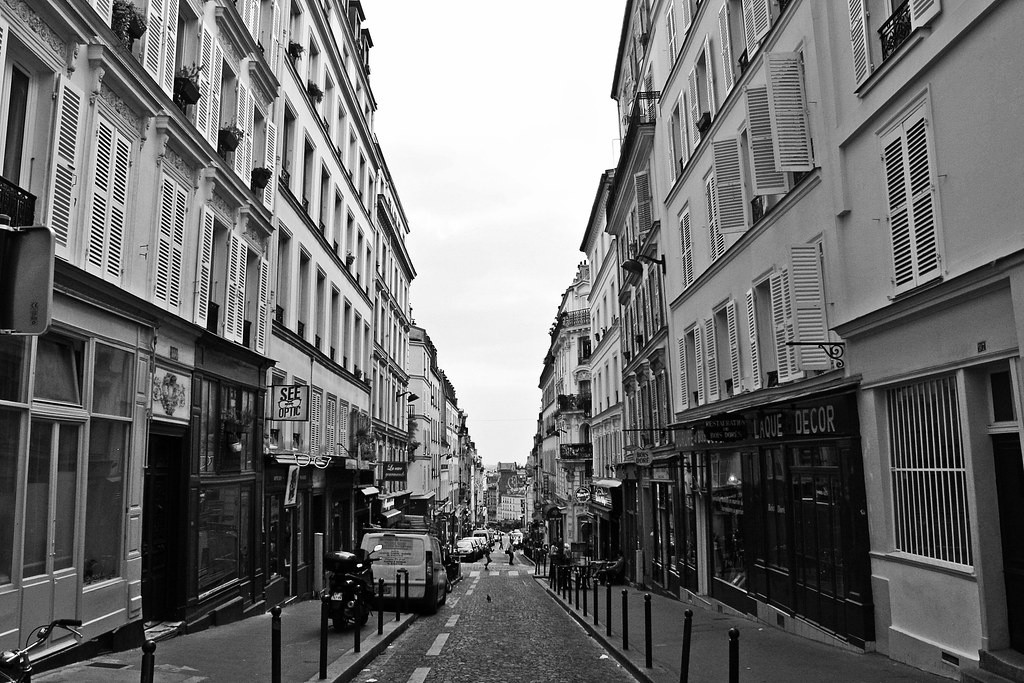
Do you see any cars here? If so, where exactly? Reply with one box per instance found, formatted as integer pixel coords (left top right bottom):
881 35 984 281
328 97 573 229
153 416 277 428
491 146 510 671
455 529 506 564
508 529 524 547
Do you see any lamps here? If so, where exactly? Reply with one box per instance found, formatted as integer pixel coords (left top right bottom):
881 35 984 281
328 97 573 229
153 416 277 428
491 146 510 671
551 429 567 437
621 253 666 277
396 391 420 403
439 453 452 461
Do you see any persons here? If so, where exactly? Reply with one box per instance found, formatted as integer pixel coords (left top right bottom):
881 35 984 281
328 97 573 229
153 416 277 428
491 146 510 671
499 533 503 550
507 540 515 565
484 544 494 570
606 551 625 585
550 537 572 559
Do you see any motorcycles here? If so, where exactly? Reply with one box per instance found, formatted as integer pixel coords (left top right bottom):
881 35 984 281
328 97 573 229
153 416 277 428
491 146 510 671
320 544 382 633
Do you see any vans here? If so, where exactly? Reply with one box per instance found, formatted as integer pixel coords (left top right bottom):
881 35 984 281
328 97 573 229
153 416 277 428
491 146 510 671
360 529 448 617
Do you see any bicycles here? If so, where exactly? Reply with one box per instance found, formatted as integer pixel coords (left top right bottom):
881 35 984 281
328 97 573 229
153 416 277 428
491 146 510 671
0 618 85 683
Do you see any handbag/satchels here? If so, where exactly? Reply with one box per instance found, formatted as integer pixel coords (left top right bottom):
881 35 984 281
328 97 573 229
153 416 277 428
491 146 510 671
505 550 510 554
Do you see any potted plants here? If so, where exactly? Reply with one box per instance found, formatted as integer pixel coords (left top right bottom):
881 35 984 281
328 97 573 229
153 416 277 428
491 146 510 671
251 167 272 189
227 410 252 452
218 121 245 152
174 60 205 105
112 0 148 45
